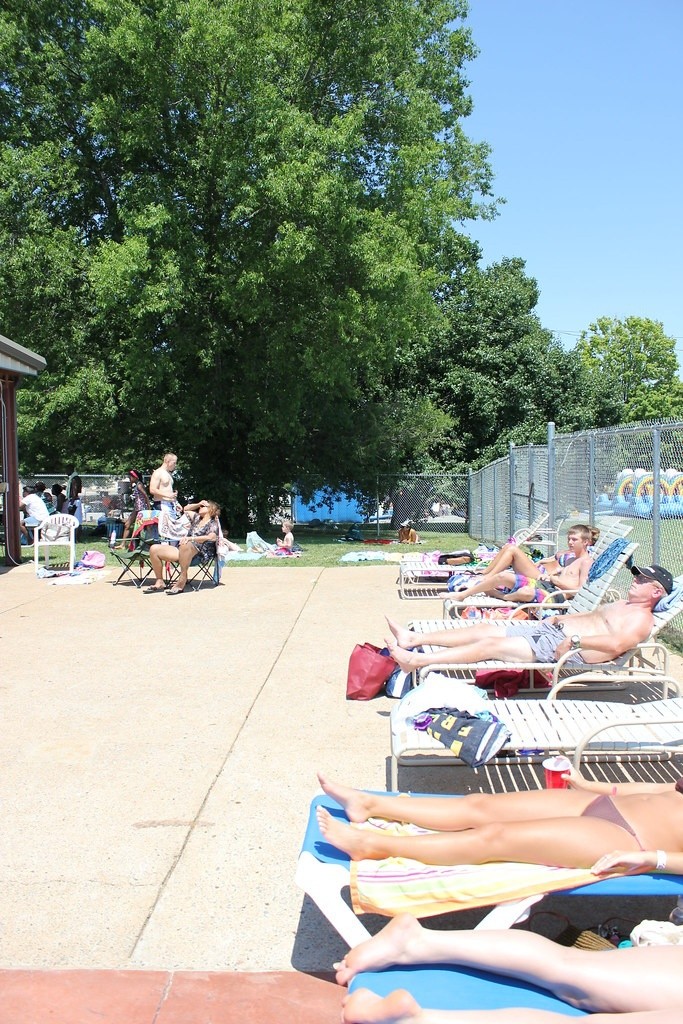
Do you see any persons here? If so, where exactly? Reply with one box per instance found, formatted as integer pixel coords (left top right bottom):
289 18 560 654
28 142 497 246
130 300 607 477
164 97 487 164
19 482 69 546
222 529 244 552
114 470 150 550
143 499 220 595
477 525 600 579
399 521 420 544
316 765 683 875
335 913 683 1024
150 453 183 547
439 524 594 609
276 521 294 555
385 564 673 674
81 492 126 522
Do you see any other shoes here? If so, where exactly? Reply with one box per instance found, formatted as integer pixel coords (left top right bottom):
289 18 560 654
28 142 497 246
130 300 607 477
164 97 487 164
114 546 125 549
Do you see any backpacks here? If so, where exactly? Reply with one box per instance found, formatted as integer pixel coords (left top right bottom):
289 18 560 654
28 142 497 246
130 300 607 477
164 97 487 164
343 522 365 542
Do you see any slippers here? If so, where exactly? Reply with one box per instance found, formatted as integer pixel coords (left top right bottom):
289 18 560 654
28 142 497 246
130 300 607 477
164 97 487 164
164 587 183 594
143 585 165 593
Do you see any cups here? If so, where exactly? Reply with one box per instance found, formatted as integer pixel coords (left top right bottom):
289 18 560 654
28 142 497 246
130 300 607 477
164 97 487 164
542 758 572 789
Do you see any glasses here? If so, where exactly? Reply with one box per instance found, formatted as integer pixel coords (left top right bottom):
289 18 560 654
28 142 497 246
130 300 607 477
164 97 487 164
631 574 660 589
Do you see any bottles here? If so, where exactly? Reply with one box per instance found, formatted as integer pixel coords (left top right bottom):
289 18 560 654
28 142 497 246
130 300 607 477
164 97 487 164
110 530 116 544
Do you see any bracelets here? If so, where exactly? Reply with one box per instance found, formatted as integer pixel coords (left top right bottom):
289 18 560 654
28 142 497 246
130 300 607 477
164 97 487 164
612 783 618 794
656 850 666 870
191 537 194 543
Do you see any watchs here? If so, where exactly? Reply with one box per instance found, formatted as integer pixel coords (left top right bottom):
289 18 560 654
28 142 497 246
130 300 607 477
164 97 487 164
572 635 581 648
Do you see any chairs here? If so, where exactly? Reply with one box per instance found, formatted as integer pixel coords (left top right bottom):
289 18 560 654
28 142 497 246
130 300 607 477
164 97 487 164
292 507 683 1015
34 514 80 574
108 514 220 591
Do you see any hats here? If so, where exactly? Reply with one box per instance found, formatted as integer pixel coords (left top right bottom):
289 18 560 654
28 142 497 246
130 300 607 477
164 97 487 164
631 564 673 595
400 520 413 529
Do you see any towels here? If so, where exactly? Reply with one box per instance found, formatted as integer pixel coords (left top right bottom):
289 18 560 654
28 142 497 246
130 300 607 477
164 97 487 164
405 706 513 768
244 531 299 561
472 667 557 699
420 551 498 578
587 538 635 581
652 581 683 611
75 550 105 569
157 506 225 586
348 813 637 919
447 571 554 602
508 622 583 665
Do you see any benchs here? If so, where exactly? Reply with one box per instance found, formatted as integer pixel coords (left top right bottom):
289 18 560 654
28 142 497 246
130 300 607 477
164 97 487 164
26 525 42 546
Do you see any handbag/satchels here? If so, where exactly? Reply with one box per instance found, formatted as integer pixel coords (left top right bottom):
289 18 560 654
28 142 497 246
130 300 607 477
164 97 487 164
345 642 398 702
